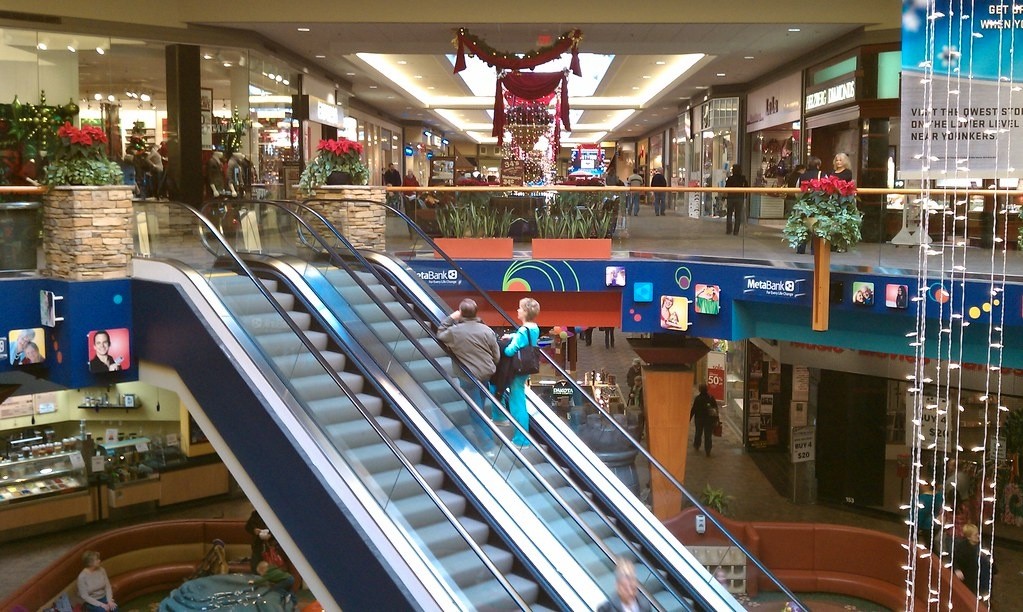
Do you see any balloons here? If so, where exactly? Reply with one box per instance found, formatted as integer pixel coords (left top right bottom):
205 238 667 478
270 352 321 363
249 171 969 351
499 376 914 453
554 325 589 343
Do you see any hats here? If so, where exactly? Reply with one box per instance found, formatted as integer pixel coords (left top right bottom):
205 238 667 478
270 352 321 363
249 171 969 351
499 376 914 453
632 358 640 366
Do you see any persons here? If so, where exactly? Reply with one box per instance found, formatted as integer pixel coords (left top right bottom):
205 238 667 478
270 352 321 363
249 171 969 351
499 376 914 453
852 286 874 305
77 550 120 612
122 154 141 198
403 169 420 238
908 473 998 609
477 174 487 182
580 327 614 349
245 509 299 608
690 382 719 457
207 148 254 240
786 153 852 255
625 356 646 440
597 556 652 612
10 330 44 365
896 286 906 308
606 167 643 216
696 285 719 314
384 163 402 213
436 298 502 457
89 330 122 373
651 167 666 216
145 141 169 198
661 296 681 329
490 297 540 449
725 163 749 234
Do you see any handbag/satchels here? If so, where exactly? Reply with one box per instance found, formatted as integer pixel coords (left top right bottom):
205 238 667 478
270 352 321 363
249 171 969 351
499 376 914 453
260 540 284 570
704 395 719 417
712 416 722 437
628 388 633 406
53 593 73 612
506 326 540 376
754 136 795 198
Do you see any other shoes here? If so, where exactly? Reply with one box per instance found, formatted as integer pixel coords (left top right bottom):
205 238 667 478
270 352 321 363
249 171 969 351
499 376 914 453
484 450 495 458
706 451 710 457
694 448 699 452
510 441 530 450
489 419 510 427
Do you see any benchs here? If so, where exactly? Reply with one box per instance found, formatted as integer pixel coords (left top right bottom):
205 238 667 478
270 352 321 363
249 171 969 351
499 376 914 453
741 521 989 612
0 518 302 612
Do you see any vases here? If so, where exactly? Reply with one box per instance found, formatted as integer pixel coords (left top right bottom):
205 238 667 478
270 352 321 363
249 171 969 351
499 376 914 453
326 171 354 185
804 217 833 235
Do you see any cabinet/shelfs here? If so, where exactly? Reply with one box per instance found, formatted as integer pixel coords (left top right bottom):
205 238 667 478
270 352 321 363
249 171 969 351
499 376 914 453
0 437 189 545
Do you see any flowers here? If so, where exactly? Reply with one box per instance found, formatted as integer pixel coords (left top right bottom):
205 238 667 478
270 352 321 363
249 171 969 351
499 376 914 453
299 137 370 196
41 119 124 185
781 175 866 254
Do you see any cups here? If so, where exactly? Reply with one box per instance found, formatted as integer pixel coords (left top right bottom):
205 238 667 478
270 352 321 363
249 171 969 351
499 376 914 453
21 437 77 458
85 396 101 407
